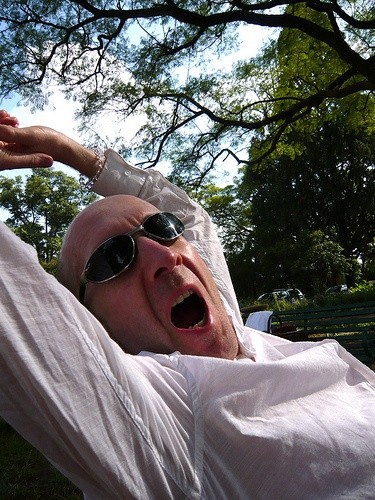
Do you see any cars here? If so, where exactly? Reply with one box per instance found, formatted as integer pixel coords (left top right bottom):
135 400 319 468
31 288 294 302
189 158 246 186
257 287 306 303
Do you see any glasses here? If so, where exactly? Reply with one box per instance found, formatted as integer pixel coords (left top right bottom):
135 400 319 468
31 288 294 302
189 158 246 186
67 211 186 303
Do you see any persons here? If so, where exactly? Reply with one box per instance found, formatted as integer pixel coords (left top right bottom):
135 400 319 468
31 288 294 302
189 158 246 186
0 109 375 500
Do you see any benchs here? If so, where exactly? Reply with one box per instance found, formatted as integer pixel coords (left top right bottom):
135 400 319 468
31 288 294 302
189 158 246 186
241 302 375 364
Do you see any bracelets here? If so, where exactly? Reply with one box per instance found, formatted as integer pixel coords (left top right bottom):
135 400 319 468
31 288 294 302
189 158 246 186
79 150 103 190
